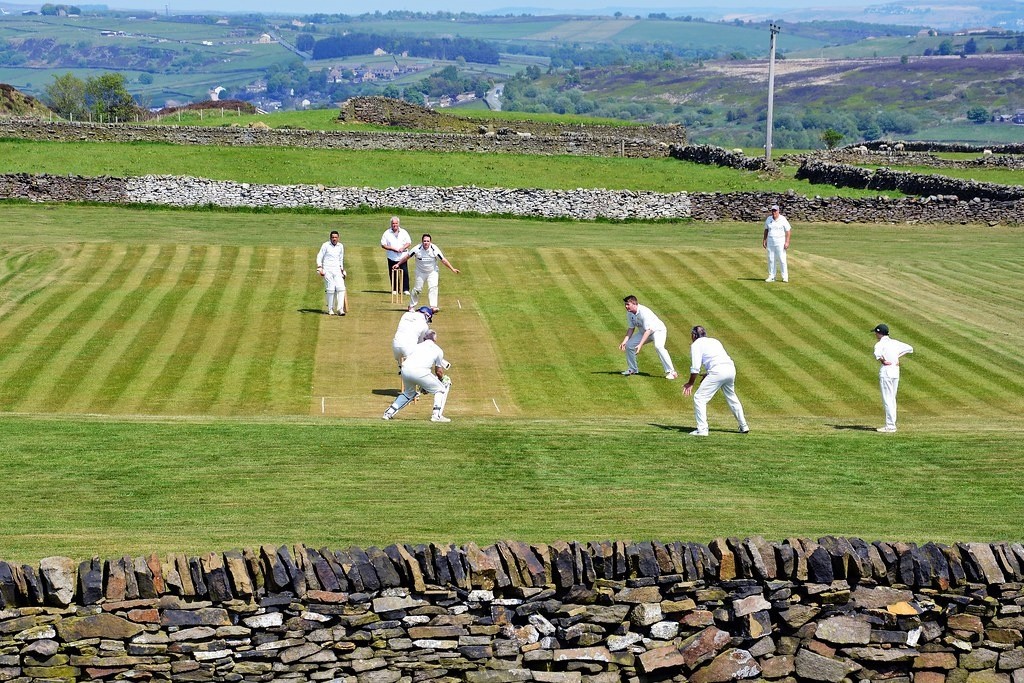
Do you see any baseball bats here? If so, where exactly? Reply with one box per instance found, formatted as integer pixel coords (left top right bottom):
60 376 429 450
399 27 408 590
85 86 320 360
343 274 349 313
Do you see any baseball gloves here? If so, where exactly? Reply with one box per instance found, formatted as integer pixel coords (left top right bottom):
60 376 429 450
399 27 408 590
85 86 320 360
419 378 443 395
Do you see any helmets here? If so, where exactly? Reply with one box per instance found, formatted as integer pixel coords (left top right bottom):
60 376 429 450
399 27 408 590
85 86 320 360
418 306 432 322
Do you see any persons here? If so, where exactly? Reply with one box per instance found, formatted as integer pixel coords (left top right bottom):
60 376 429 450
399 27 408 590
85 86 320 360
382 330 451 422
381 217 411 295
391 307 432 361
316 231 346 316
680 326 749 436
619 295 677 379
763 206 791 282
392 234 460 312
870 324 913 432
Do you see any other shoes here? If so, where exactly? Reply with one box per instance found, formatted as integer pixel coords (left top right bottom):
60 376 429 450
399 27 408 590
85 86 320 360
403 291 410 295
782 278 788 283
431 306 439 314
876 425 897 432
764 277 777 283
337 308 345 316
382 412 392 420
738 424 749 433
393 291 397 295
408 306 415 312
666 372 677 379
431 414 451 423
621 368 638 374
689 429 708 436
328 308 334 315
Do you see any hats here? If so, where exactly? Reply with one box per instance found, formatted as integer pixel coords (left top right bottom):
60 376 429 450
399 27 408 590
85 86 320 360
870 324 889 335
771 205 780 211
